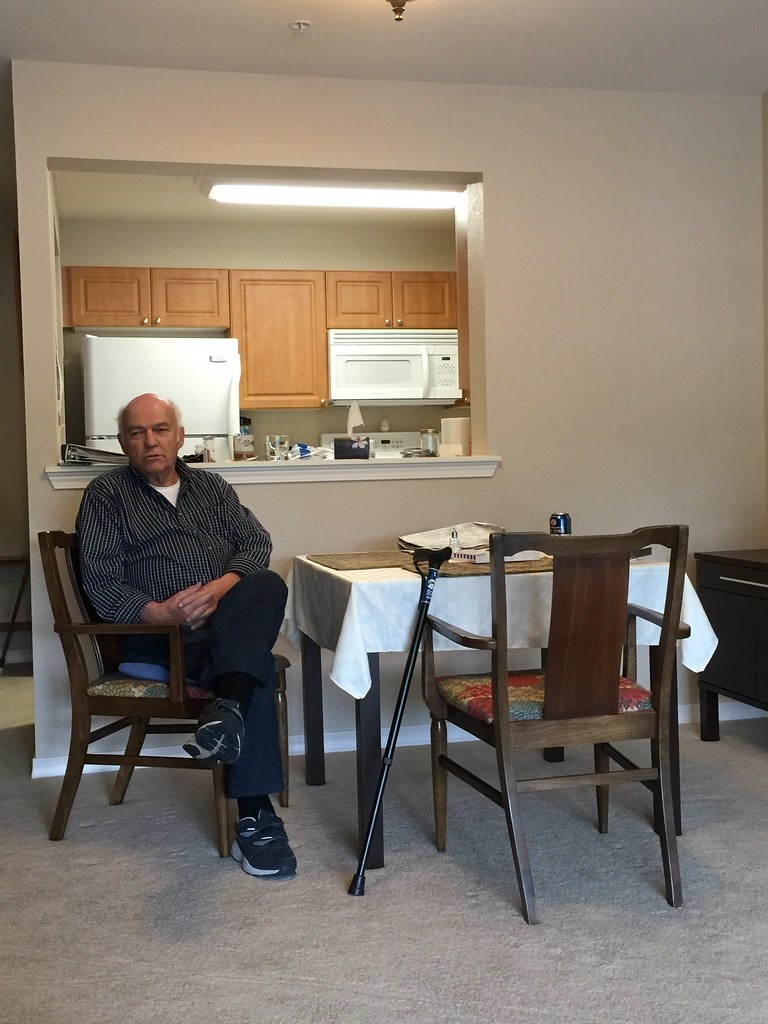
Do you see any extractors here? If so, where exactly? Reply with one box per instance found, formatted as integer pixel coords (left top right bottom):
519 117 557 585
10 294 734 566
332 399 456 405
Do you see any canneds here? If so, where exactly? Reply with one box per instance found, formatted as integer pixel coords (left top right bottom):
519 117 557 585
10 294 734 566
550 512 571 537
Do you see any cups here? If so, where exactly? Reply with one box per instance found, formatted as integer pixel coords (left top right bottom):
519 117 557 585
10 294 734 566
235 435 255 461
265 435 289 461
334 436 370 460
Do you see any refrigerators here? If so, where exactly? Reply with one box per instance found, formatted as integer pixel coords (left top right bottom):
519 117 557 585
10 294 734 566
82 335 241 461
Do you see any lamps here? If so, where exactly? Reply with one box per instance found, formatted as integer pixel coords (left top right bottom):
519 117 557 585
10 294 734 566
200 175 466 210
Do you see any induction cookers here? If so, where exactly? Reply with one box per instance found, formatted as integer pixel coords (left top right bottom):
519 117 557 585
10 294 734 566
320 432 441 459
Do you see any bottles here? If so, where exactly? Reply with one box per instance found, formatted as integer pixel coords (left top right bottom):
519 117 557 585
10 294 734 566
240 417 253 435
421 429 439 458
450 531 461 558
195 437 215 463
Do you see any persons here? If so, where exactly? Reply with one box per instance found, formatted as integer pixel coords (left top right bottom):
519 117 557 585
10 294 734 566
75 393 298 879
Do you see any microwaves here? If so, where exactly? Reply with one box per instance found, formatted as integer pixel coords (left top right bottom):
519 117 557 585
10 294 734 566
326 329 463 399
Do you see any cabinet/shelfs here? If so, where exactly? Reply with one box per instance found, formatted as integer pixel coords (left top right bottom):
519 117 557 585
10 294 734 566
694 548 768 741
60 266 230 333
325 270 457 329
228 269 329 411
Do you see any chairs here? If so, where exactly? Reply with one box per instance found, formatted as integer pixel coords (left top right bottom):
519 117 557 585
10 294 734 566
421 524 684 924
39 530 291 859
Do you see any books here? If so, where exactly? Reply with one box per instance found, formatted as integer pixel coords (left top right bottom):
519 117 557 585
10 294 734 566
58 444 129 466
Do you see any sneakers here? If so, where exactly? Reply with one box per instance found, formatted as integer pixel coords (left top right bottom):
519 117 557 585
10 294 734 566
183 700 246 768
230 809 297 878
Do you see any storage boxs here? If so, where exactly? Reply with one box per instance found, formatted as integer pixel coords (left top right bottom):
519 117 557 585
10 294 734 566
454 553 490 563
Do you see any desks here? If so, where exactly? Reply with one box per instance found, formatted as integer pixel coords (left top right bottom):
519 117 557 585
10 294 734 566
279 551 720 870
